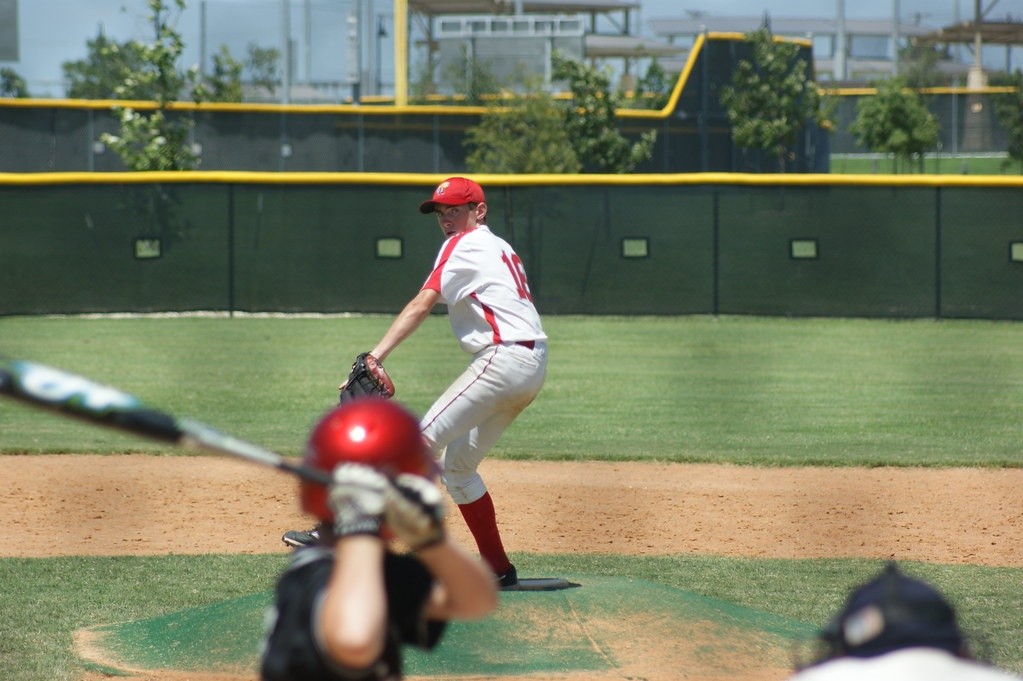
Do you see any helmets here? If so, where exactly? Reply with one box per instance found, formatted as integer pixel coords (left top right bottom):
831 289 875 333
303 399 439 521
820 574 962 656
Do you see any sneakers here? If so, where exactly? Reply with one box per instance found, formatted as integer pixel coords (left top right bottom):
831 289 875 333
494 564 517 587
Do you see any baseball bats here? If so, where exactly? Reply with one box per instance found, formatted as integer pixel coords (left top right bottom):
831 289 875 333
0 360 331 483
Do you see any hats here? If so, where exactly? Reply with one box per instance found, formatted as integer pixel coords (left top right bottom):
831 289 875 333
419 177 486 214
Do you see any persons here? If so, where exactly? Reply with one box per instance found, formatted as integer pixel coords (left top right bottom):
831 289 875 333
283 178 548 590
257 400 499 681
787 560 1023 681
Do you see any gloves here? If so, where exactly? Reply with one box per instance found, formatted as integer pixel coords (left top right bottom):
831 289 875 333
387 473 445 551
326 463 387 538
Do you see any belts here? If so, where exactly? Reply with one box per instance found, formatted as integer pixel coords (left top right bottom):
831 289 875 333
515 340 535 351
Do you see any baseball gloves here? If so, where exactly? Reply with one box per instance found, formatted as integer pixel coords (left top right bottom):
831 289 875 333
340 352 395 405
